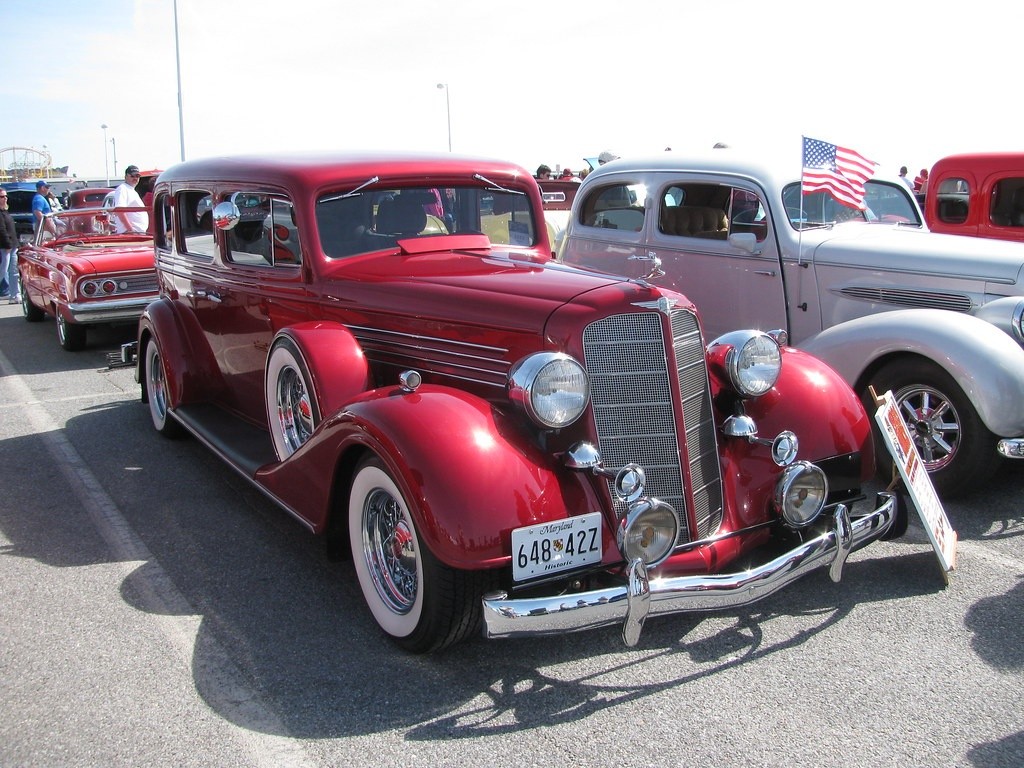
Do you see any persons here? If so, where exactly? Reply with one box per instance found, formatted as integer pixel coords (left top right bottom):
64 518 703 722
416 189 456 223
32 181 53 233
898 167 928 192
598 152 632 207
537 165 591 182
0 189 22 304
115 165 148 233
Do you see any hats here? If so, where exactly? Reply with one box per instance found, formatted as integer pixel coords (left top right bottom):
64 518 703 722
563 168 573 175
125 165 141 176
598 150 622 166
36 180 52 188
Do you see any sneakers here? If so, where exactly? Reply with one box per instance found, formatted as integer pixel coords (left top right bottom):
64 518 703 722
9 298 22 304
0 293 11 299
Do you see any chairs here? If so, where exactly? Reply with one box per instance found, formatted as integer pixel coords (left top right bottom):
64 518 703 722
375 198 427 245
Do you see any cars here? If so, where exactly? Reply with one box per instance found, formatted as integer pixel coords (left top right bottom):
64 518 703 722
556 150 1024 499
63 170 162 211
16 206 168 351
137 150 909 654
923 154 1023 242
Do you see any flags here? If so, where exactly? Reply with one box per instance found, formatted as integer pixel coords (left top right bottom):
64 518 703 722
802 137 875 209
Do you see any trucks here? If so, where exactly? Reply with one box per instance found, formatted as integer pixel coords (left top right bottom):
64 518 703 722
0 182 57 245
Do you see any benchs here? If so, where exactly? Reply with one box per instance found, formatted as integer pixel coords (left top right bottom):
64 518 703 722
194 209 366 265
658 205 730 239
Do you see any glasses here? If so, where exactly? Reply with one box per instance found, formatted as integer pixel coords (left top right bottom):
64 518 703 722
46 187 49 189
0 195 7 198
131 174 140 177
546 174 550 177
